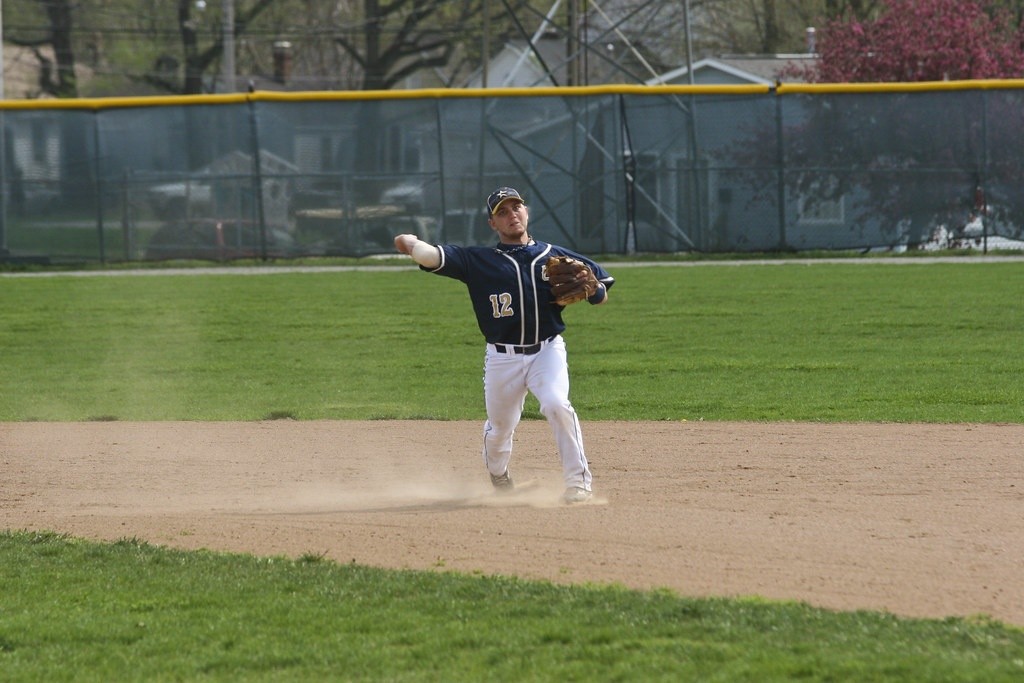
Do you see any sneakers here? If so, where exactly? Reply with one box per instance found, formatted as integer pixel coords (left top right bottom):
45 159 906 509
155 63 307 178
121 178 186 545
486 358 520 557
564 487 607 506
488 465 514 492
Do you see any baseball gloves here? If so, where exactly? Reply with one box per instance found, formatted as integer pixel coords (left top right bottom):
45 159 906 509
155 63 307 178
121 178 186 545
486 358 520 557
544 254 599 307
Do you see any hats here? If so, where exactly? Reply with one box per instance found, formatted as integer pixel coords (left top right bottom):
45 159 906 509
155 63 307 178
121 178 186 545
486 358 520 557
486 187 524 218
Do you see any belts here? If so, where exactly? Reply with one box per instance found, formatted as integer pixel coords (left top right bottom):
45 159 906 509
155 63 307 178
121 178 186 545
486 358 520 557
495 334 558 355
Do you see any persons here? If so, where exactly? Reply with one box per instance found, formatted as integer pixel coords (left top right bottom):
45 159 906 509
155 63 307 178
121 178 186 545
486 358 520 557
394 186 614 502
